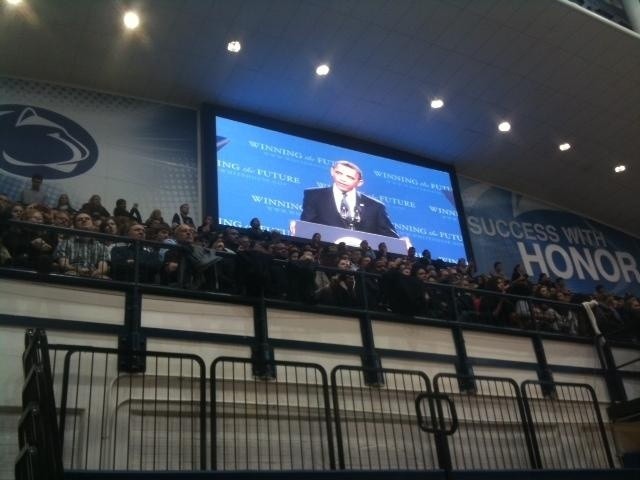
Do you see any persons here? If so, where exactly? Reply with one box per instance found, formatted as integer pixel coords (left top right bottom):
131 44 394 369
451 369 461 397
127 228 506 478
303 159 402 240
326 238 639 342
1 194 331 305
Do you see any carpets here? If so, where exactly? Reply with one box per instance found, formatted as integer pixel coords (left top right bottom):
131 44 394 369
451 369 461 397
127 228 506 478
340 193 349 216
